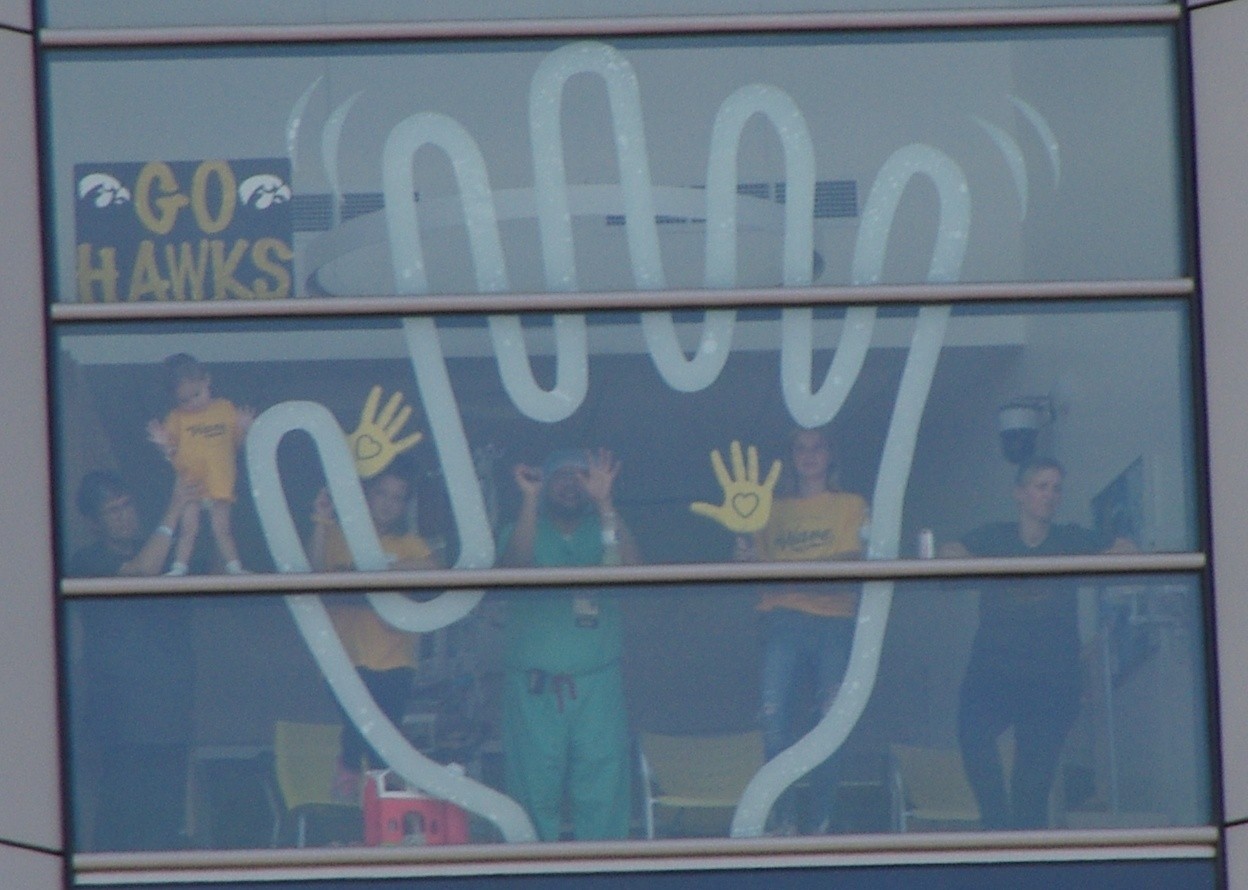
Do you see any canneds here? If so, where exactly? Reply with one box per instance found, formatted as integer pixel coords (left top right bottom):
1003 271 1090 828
917 529 936 559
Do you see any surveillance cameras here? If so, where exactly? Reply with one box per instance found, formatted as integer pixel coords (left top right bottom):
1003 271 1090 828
1001 406 1036 465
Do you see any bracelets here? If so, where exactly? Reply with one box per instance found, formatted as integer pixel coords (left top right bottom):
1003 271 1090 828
154 524 175 537
598 507 619 519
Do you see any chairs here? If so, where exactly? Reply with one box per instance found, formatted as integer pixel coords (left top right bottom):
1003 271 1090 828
635 732 767 839
886 726 1015 831
268 721 351 849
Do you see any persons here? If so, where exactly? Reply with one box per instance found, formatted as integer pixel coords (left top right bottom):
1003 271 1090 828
940 454 1144 832
489 436 655 850
304 453 451 845
734 425 890 838
69 465 210 854
140 353 260 579
